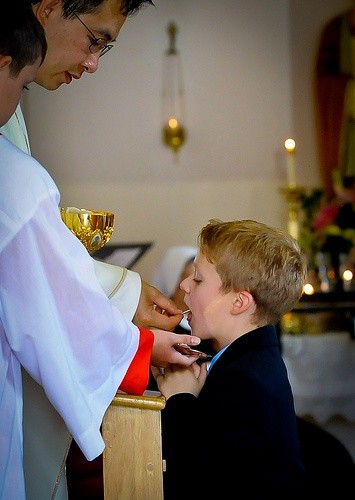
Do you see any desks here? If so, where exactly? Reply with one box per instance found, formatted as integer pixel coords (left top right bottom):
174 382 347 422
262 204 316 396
102 393 166 500
282 290 355 342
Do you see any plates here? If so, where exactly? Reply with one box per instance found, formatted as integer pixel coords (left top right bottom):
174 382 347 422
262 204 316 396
174 343 213 359
